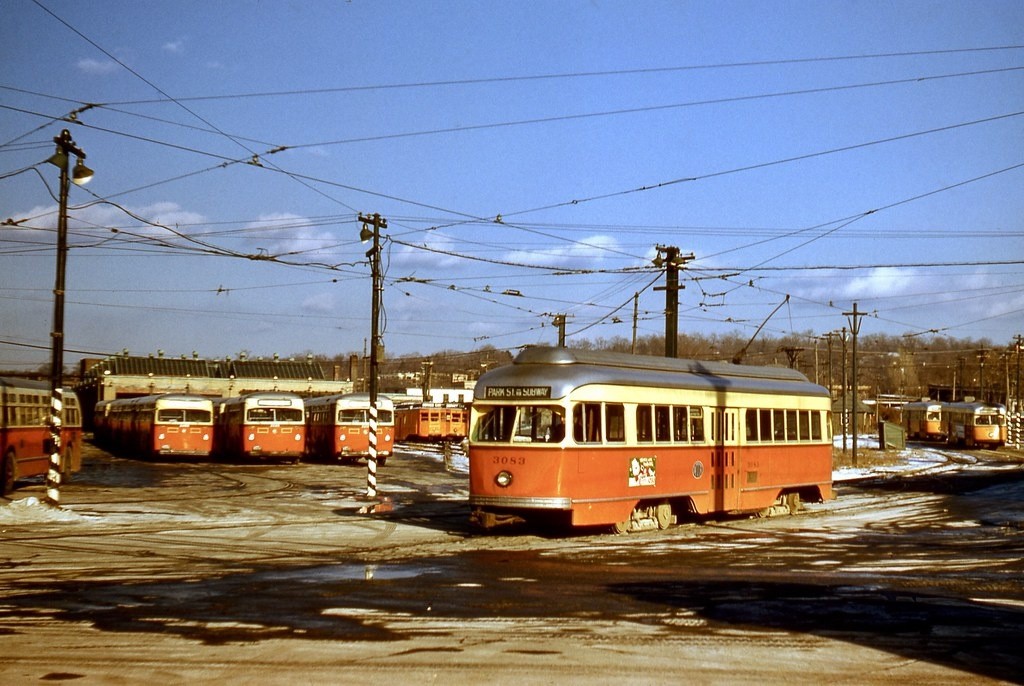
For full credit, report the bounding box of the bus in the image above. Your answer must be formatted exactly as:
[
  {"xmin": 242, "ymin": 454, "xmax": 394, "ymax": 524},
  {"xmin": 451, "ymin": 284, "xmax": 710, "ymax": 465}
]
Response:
[
  {"xmin": 219, "ymin": 391, "xmax": 306, "ymax": 464},
  {"xmin": 393, "ymin": 401, "xmax": 469, "ymax": 445},
  {"xmin": 946, "ymin": 403, "xmax": 1008, "ymax": 449},
  {"xmin": 94, "ymin": 392, "xmax": 214, "ymax": 463},
  {"xmin": 465, "ymin": 346, "xmax": 835, "ymax": 534},
  {"xmin": 901, "ymin": 400, "xmax": 949, "ymax": 441},
  {"xmin": 308, "ymin": 392, "xmax": 394, "ymax": 467},
  {"xmin": 0, "ymin": 376, "xmax": 83, "ymax": 494}
]
